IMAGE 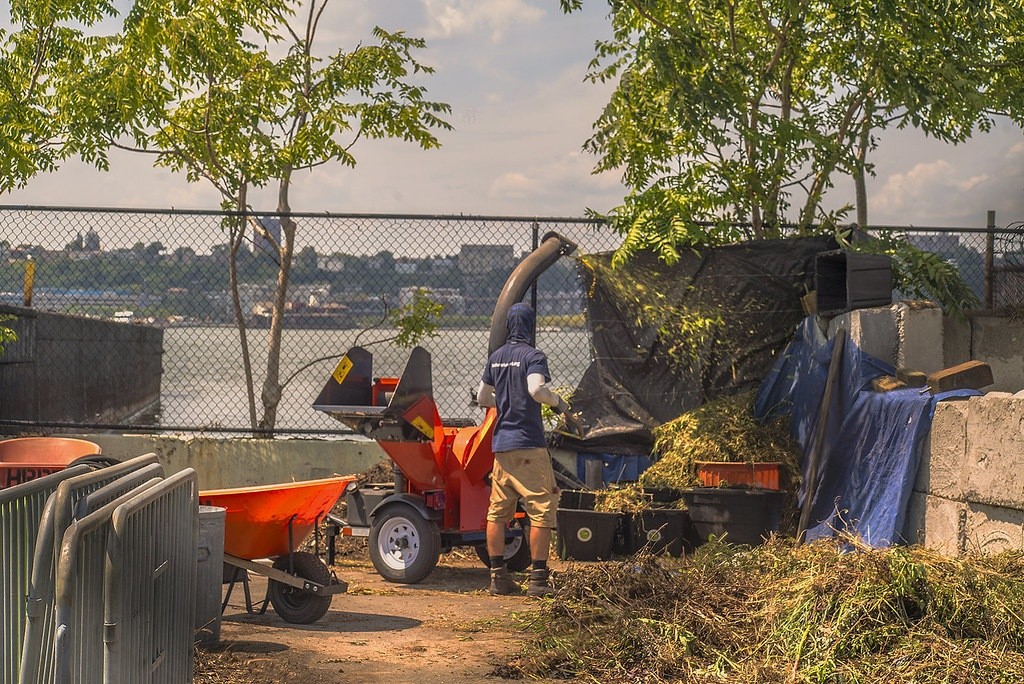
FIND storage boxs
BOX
[555,460,784,562]
[814,249,892,317]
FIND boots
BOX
[528,566,566,597]
[489,564,522,594]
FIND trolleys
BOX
[196,474,357,624]
[0,435,102,491]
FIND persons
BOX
[476,303,585,597]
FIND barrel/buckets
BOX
[624,502,686,558]
[557,489,624,562]
[193,506,226,649]
[681,485,788,549]
[642,486,679,503]
